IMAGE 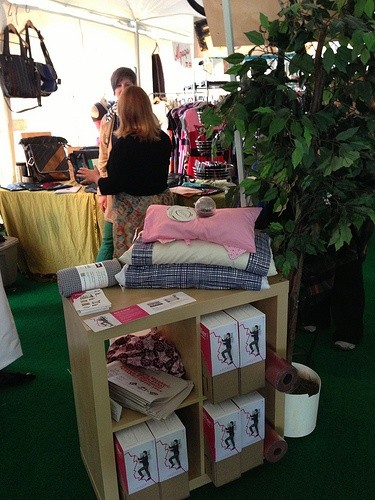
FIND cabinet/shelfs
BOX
[64,279,290,500]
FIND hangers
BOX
[150,92,214,109]
[0,20,44,47]
[152,43,160,55]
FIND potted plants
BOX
[206,1,375,437]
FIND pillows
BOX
[115,205,278,290]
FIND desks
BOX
[0,188,114,282]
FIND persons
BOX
[96,67,137,262]
[0,273,36,387]
[137,450,151,481]
[97,315,114,327]
[249,409,259,437]
[76,86,172,259]
[224,421,236,451]
[168,439,181,470]
[91,94,115,146]
[249,325,260,357]
[221,333,234,365]
[300,75,375,351]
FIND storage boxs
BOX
[115,303,266,500]
[0,236,19,286]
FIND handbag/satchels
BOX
[1,26,45,113]
[24,24,61,98]
[18,136,73,183]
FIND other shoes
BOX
[0,370,36,387]
